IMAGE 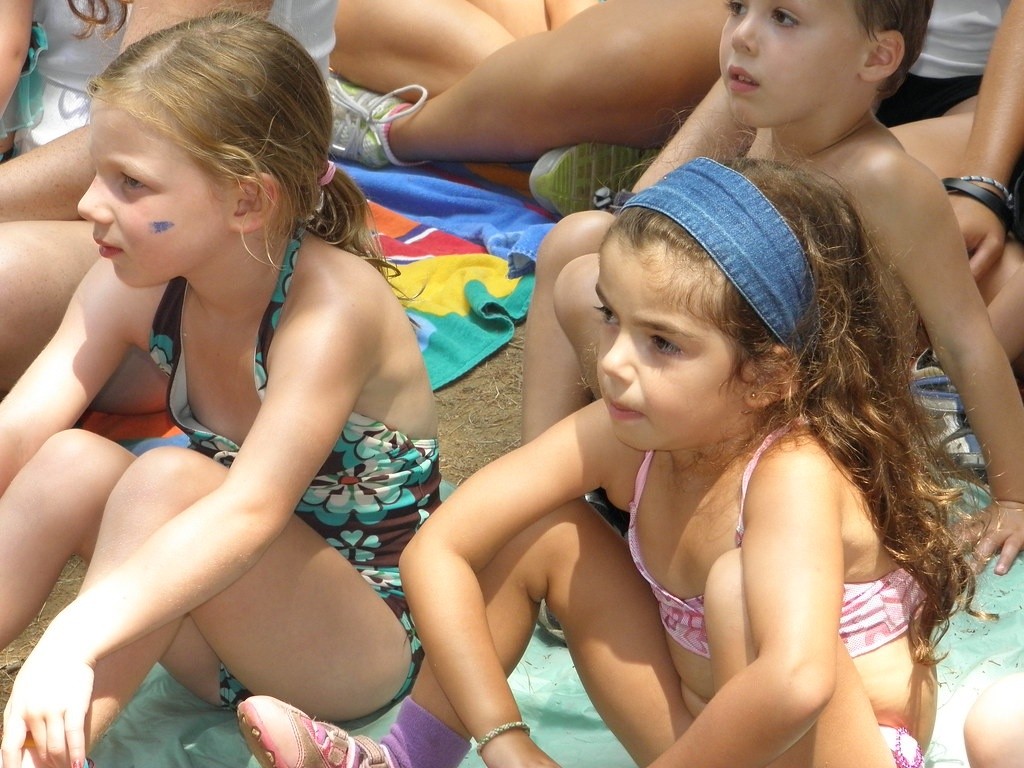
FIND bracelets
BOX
[476,722,531,757]
[940,177,1015,232]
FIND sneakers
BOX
[236,693,393,768]
[529,140,684,218]
[285,73,427,169]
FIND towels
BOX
[63,470,1024,768]
[318,151,571,403]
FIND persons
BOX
[3,0,1024,418]
[227,154,954,768]
[0,15,441,768]
[961,673,1024,768]
[522,0,1023,647]
